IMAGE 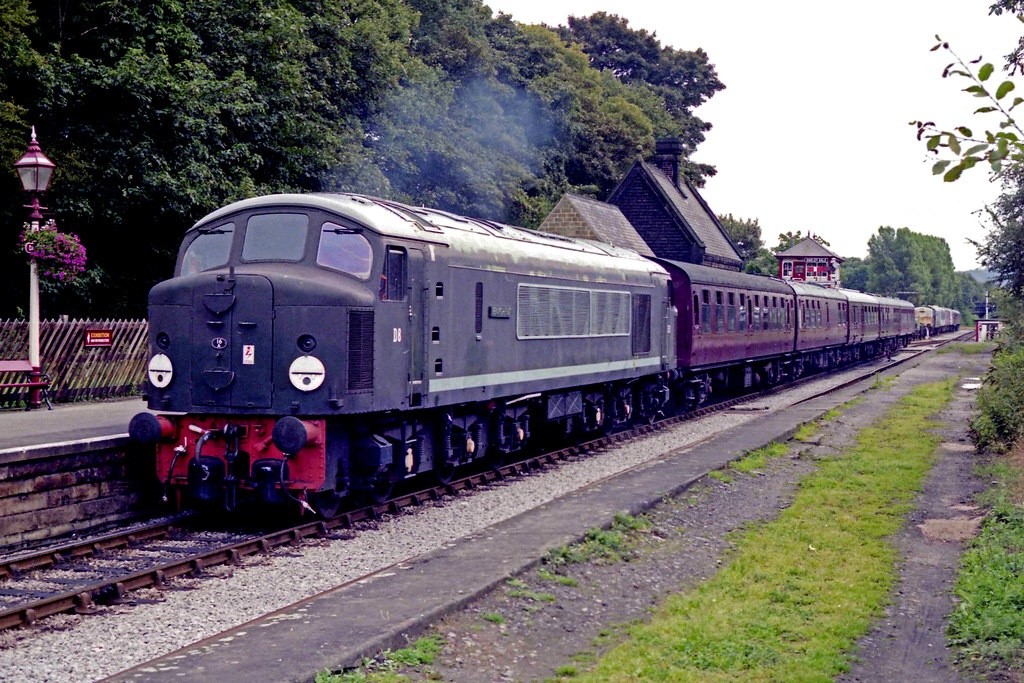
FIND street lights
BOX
[13,125,56,410]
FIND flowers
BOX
[15,218,102,305]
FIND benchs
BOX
[0,360,54,410]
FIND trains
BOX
[116,192,960,530]
